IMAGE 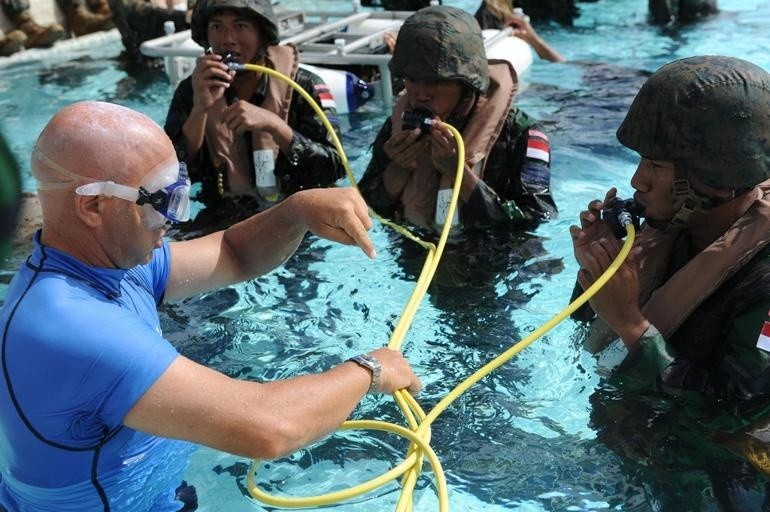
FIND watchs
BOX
[345,354,383,396]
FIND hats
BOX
[387,6,489,92]
[190,1,282,46]
[617,55,770,220]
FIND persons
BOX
[569,54,770,512]
[472,0,565,64]
[55,0,119,38]
[165,1,347,230]
[356,5,559,238]
[108,0,193,60]
[0,0,63,56]
[1,101,423,512]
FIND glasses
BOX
[75,159,192,223]
